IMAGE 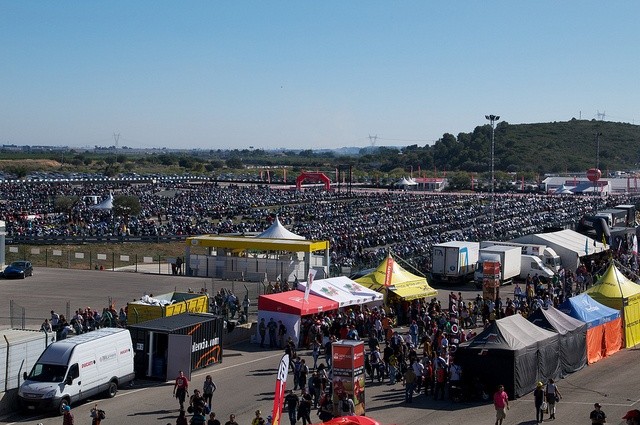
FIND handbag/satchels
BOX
[555,385,560,402]
[543,396,548,414]
[540,390,547,410]
[187,396,195,413]
[416,363,424,374]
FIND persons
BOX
[41,287,251,339]
[267,415,271,424]
[251,410,265,425]
[308,297,449,398]
[493,385,509,424]
[63,405,74,424]
[176,410,188,424]
[589,403,607,425]
[202,375,216,409]
[173,371,189,408]
[278,320,287,348]
[208,412,221,425]
[338,391,355,415]
[283,336,335,425]
[544,377,563,420]
[0,179,635,276]
[259,317,267,346]
[450,264,593,328]
[225,414,239,424]
[189,406,206,425]
[533,381,546,424]
[189,388,205,414]
[621,409,640,425]
[267,317,277,347]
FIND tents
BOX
[578,259,640,350]
[297,276,384,314]
[352,247,438,324]
[525,303,587,374]
[256,288,338,346]
[555,291,622,365]
[458,314,558,400]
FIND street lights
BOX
[485,114,500,239]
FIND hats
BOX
[63,406,70,411]
[622,410,640,419]
[347,309,352,311]
[536,382,543,386]
[194,389,200,393]
[266,416,271,419]
[303,394,312,400]
[594,403,601,407]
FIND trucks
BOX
[522,255,554,280]
[595,209,626,227]
[481,241,562,273]
[431,240,480,279]
[474,245,522,290]
[18,328,135,417]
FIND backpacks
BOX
[341,399,350,412]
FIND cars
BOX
[4,260,34,281]
